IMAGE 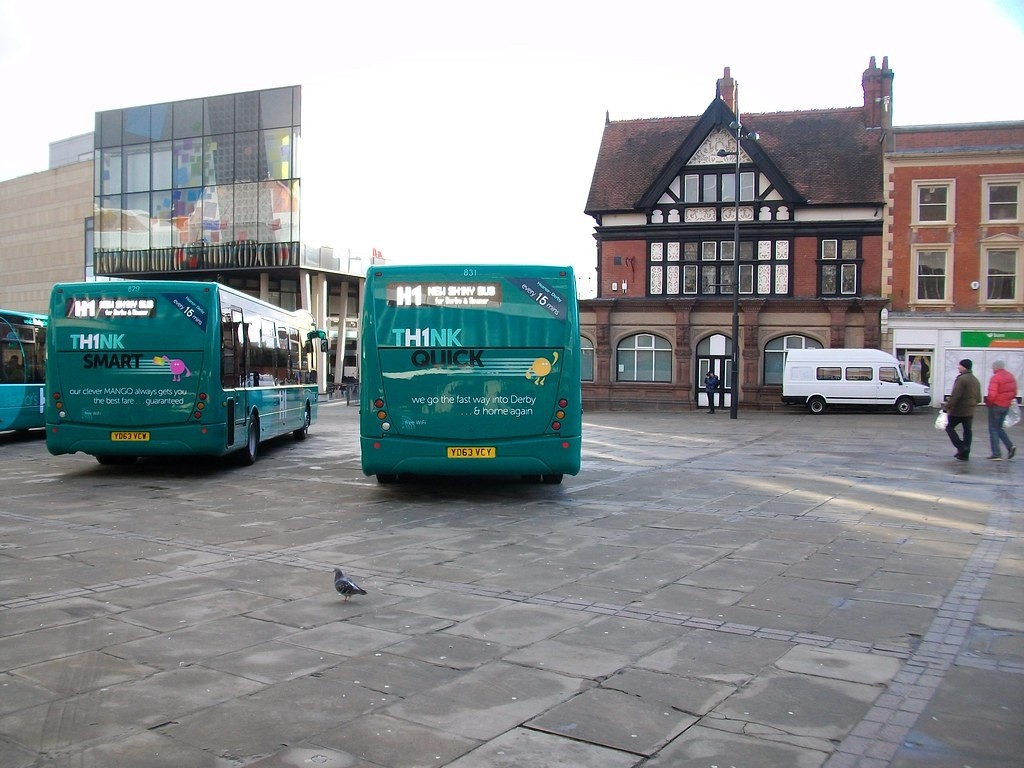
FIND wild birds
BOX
[332,566,368,603]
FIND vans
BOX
[781,346,932,416]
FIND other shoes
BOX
[1008,445,1016,459]
[954,453,969,462]
[707,410,714,414]
[986,456,1003,461]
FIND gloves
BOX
[943,409,947,413]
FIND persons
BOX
[704,371,717,414]
[987,360,1016,460]
[943,359,982,461]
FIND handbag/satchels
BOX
[935,410,948,430]
[1003,399,1021,427]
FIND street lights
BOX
[718,79,756,420]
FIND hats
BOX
[994,360,1005,368]
[708,371,713,373]
[959,359,972,370]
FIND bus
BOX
[356,265,584,485]
[0,308,47,435]
[44,280,330,466]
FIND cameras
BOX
[706,373,710,376]
